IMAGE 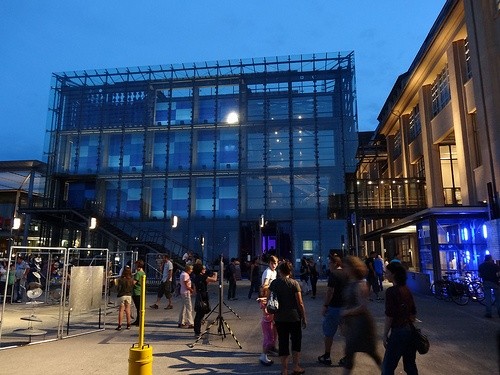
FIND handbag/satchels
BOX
[414,328,429,355]
[266,281,280,314]
[197,300,211,314]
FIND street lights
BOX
[169,215,179,261]
[199,235,206,267]
[87,217,97,247]
[257,213,265,262]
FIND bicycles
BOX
[429,270,499,307]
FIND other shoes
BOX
[228,297,239,301]
[131,321,139,325]
[188,325,194,329]
[368,298,373,302]
[117,325,121,330]
[311,296,315,298]
[127,325,131,329]
[270,348,279,356]
[318,353,332,366]
[178,323,187,328]
[484,314,492,318]
[150,304,158,309]
[195,333,205,338]
[291,367,305,375]
[337,357,346,365]
[375,297,382,302]
[260,356,274,365]
[166,305,173,310]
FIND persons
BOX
[327,249,343,260]
[342,256,382,375]
[317,256,354,368]
[248,260,261,299]
[266,260,308,375]
[224,257,241,301]
[190,263,217,340]
[114,268,133,330]
[300,256,312,295]
[182,250,198,266]
[379,262,420,375]
[275,257,295,280]
[308,256,319,299]
[178,264,194,328]
[259,284,276,366]
[363,251,415,301]
[0,255,64,303]
[263,255,280,357]
[479,254,500,319]
[132,259,145,327]
[150,255,174,310]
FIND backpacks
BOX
[224,266,232,280]
